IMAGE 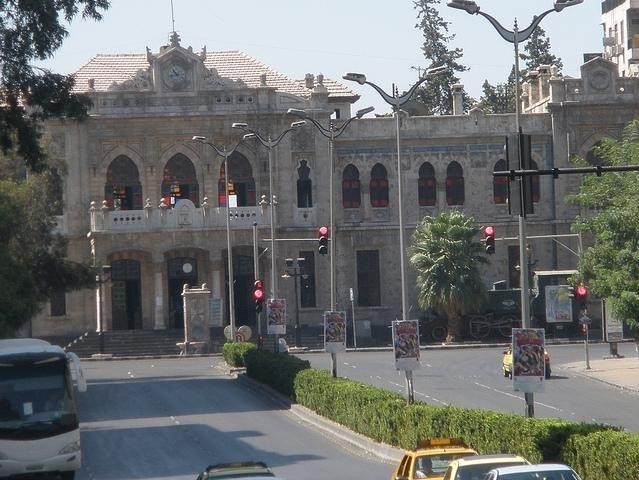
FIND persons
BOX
[279,334,289,354]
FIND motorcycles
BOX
[417,306,447,342]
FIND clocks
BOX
[167,65,186,84]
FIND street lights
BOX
[341,65,449,401]
[281,258,311,350]
[287,107,374,378]
[191,132,258,342]
[231,120,308,354]
[445,0,585,420]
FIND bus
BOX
[0,338,88,480]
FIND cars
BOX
[389,438,583,480]
[196,461,277,480]
[502,341,551,379]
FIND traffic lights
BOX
[574,284,587,300]
[484,225,495,255]
[253,279,265,312]
[319,226,328,254]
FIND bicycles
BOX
[469,310,521,340]
